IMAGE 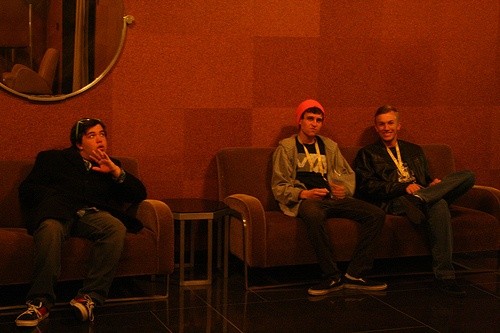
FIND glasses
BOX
[76,118,104,139]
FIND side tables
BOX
[165,198,228,287]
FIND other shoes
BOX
[397,194,427,224]
[436,279,467,297]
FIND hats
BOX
[296,99,325,125]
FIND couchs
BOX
[215,147,500,290]
[0,156,175,310]
[2,48,59,95]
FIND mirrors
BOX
[0,0,134,102]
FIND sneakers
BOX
[14,300,49,326]
[69,294,95,321]
[341,273,387,290]
[308,278,343,296]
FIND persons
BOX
[352,105,476,295]
[14,117,147,326]
[271,99,388,295]
